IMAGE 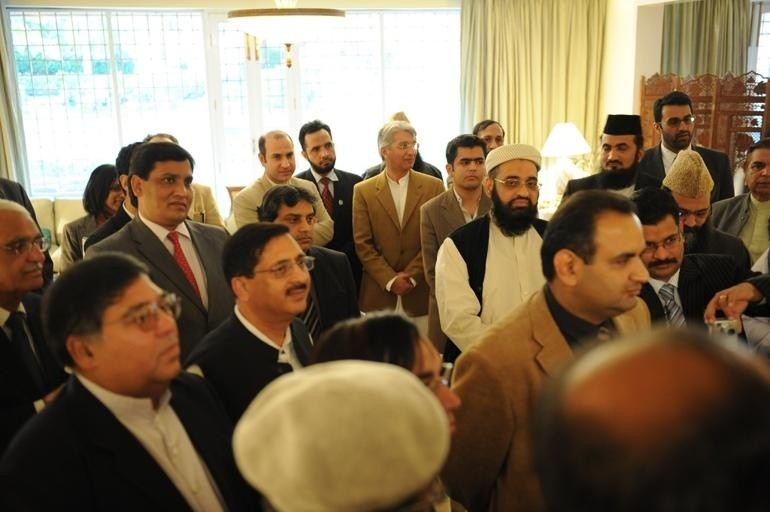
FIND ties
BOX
[319,177,335,218]
[595,326,615,346]
[166,231,203,304]
[296,293,323,346]
[658,283,688,331]
[3,311,42,381]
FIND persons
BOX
[0,92,769,512]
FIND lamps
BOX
[229,0,347,69]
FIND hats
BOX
[485,144,542,175]
[603,115,641,134]
[663,150,714,197]
[233,360,451,512]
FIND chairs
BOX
[30,198,89,273]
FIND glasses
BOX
[643,231,681,250]
[112,185,124,191]
[103,293,181,331]
[657,116,695,127]
[248,256,314,277]
[389,144,418,150]
[278,217,318,225]
[679,206,713,218]
[494,177,542,190]
[426,362,455,389]
[1,236,51,256]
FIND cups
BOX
[708,320,738,352]
